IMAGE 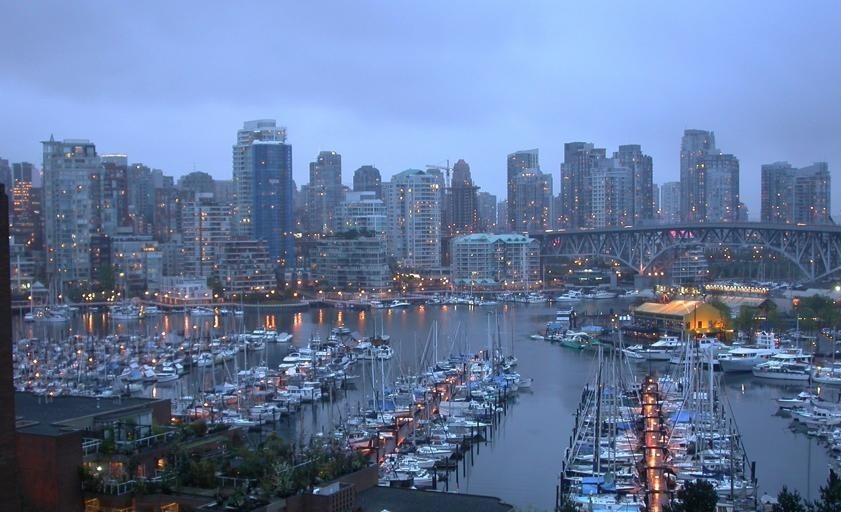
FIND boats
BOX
[717,328,783,374]
[498,280,548,303]
[370,300,385,309]
[13,306,322,430]
[752,349,841,454]
[554,284,638,301]
[421,280,497,306]
[389,300,412,308]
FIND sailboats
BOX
[542,300,760,512]
[323,306,532,488]
